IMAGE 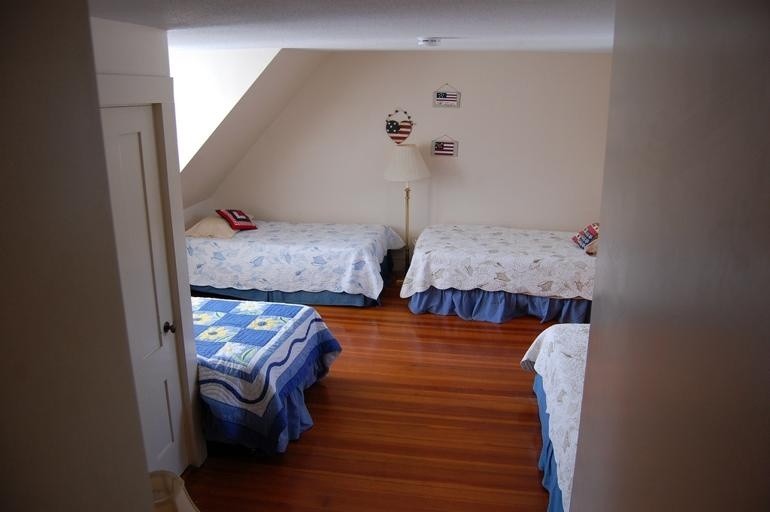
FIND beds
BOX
[186,219,406,307]
[191,295,342,452]
[520,322,590,512]
[400,224,597,324]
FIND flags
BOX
[436,92,457,105]
[434,142,454,155]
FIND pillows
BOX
[184,213,255,238]
[571,223,599,249]
[585,238,598,255]
[215,208,257,229]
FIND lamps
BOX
[385,144,431,284]
